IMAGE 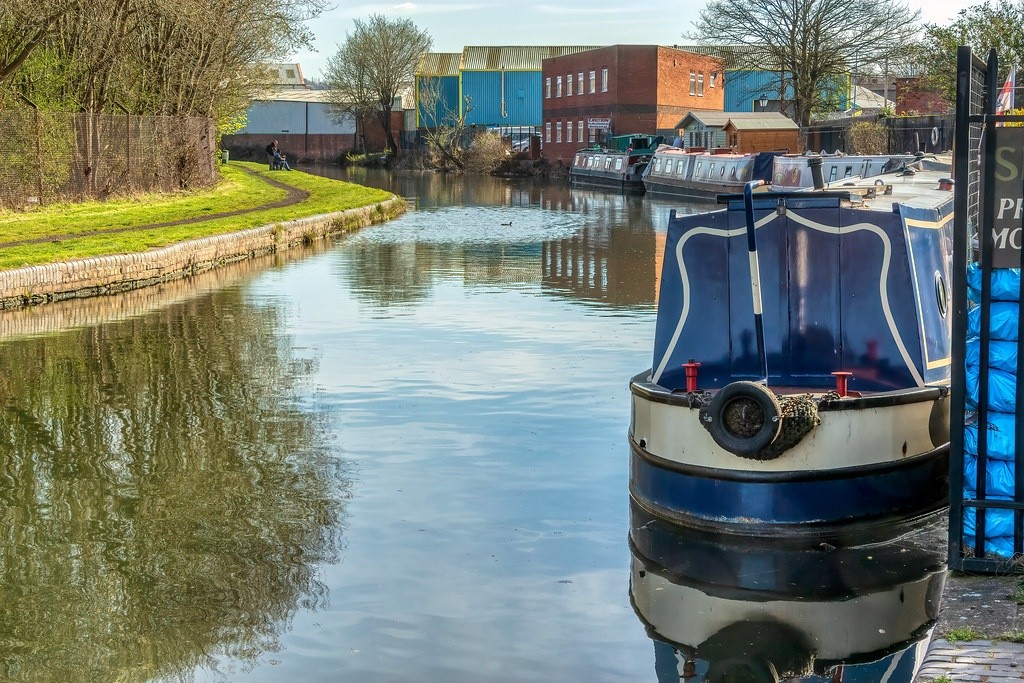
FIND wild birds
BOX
[501,221,513,227]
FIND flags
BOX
[995,72,1013,120]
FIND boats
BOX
[565,133,951,683]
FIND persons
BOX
[266,139,279,170]
[274,149,293,171]
